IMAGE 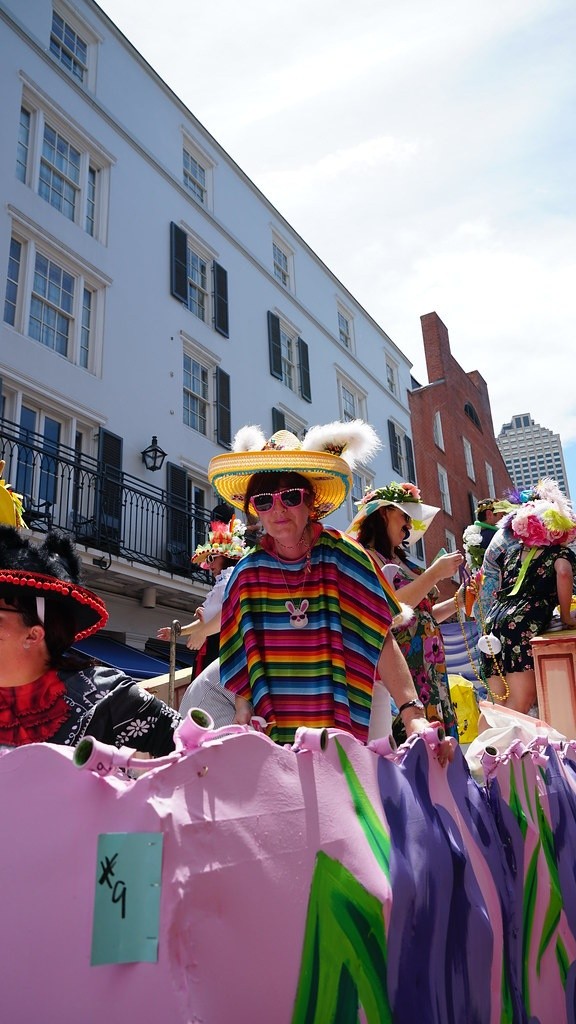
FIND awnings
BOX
[70,632,179,680]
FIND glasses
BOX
[205,554,219,564]
[249,489,309,513]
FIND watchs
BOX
[400,699,424,712]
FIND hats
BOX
[0,460,109,643]
[345,481,441,549]
[207,418,379,518]
[191,514,250,564]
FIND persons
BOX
[178,658,237,741]
[156,522,246,680]
[218,430,454,771]
[345,482,477,744]
[0,525,181,772]
[211,502,260,533]
[466,480,576,715]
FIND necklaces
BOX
[454,583,509,699]
[275,548,309,628]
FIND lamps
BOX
[141,436,168,472]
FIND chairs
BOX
[68,513,120,556]
[14,491,54,532]
[167,541,207,579]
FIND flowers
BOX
[353,482,422,510]
[462,525,488,570]
